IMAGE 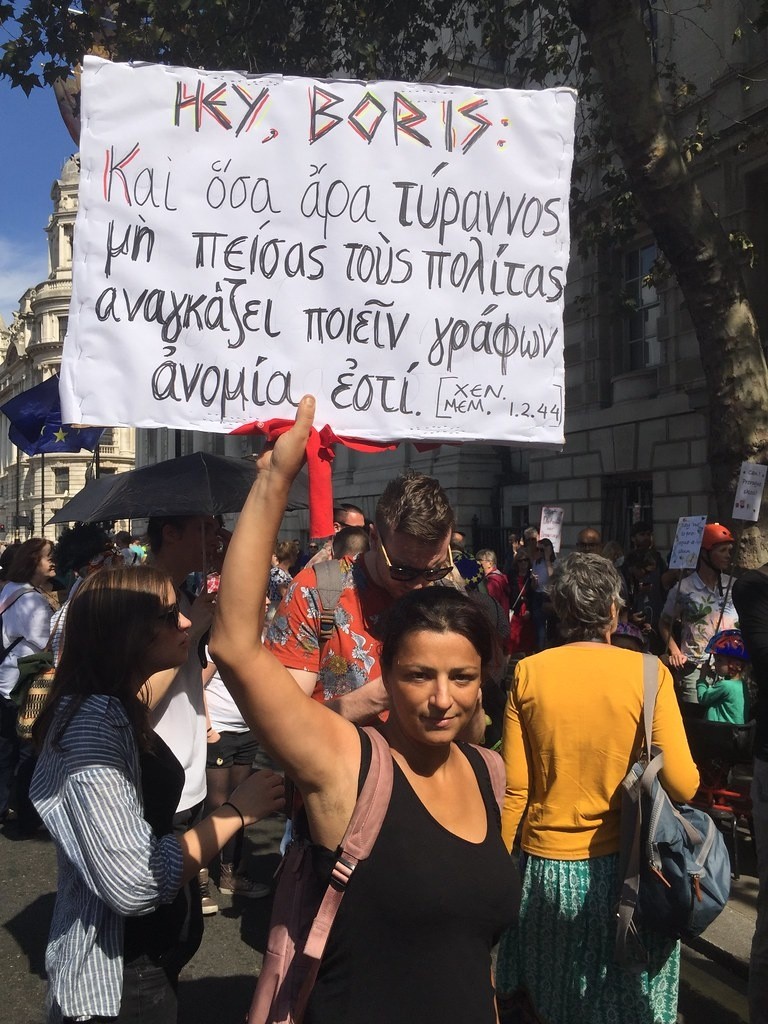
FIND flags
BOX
[0,370,107,458]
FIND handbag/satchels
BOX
[14,608,64,741]
[540,601,557,617]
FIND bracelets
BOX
[222,802,244,832]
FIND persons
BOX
[733,564,768,1024]
[450,520,758,724]
[500,552,703,1024]
[207,393,522,1024]
[0,503,372,913]
[29,562,288,1024]
[275,468,487,746]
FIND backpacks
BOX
[612,653,731,974]
[244,725,506,1024]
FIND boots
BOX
[219,862,272,900]
[198,868,219,914]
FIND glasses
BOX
[375,528,453,581]
[517,558,529,563]
[642,567,654,576]
[525,537,537,542]
[575,543,602,551]
[536,547,544,552]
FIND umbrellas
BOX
[42,449,349,528]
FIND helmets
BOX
[702,523,735,550]
[704,629,752,662]
[612,620,644,651]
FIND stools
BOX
[705,808,742,881]
